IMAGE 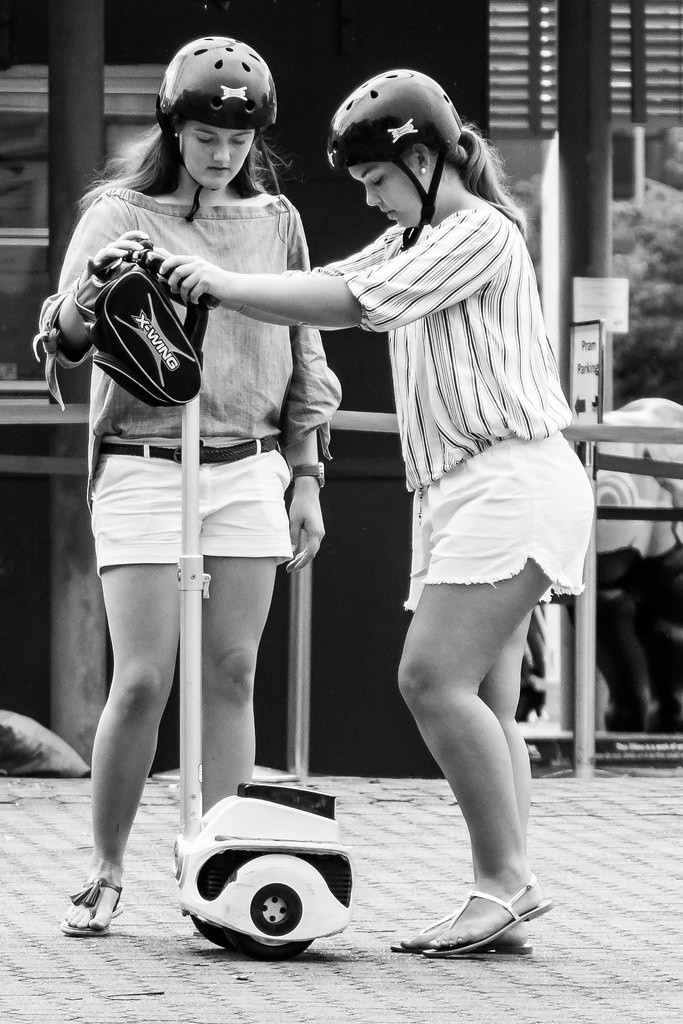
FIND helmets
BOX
[156,35,277,128]
[326,68,463,170]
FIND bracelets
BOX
[237,305,247,313]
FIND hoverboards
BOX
[74,240,354,963]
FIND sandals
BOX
[390,908,533,955]
[61,877,124,935]
[423,873,554,962]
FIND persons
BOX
[133,68,595,958]
[34,36,342,936]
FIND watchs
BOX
[290,462,325,488]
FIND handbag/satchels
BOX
[74,252,209,406]
